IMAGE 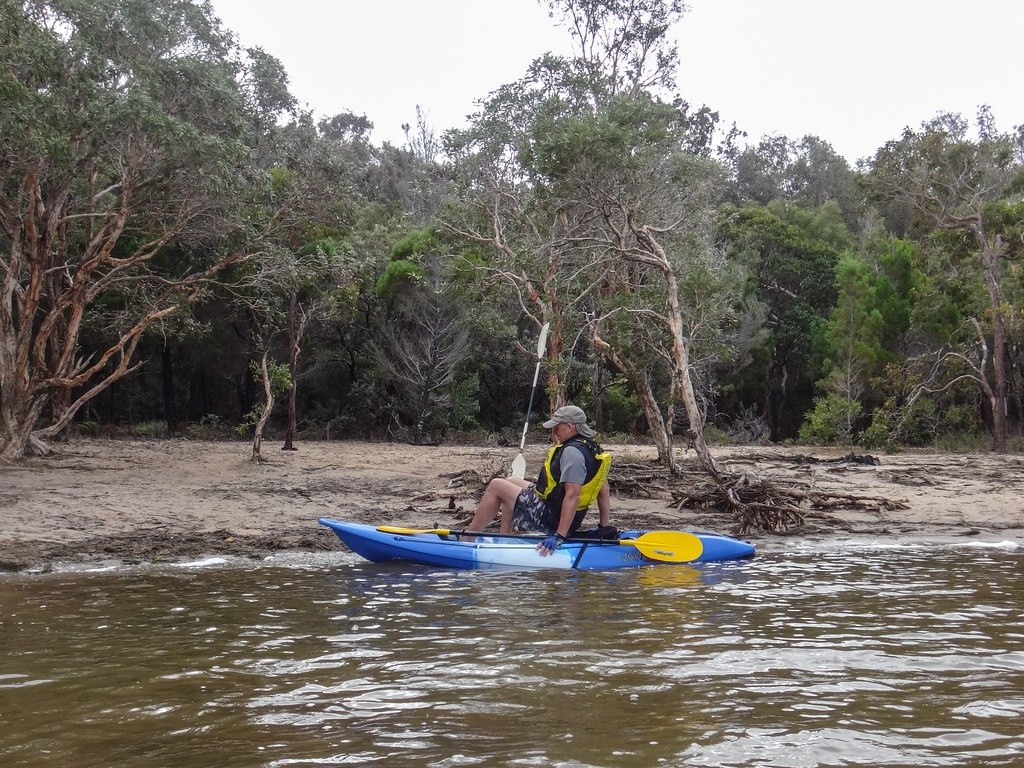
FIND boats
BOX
[318,518,757,570]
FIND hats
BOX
[542,405,597,438]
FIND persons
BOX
[433,405,612,558]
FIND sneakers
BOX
[433,523,459,542]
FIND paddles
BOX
[506,321,551,480]
[373,524,705,563]
